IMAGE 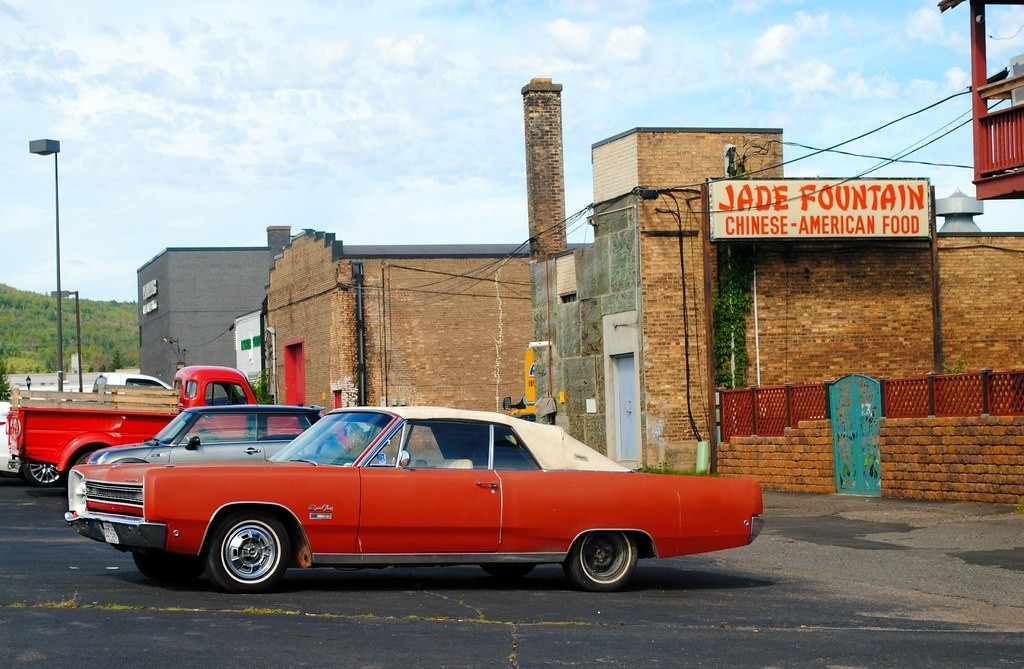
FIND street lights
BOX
[28,137,72,392]
[48,290,88,391]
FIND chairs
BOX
[435,459,474,469]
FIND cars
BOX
[85,404,353,474]
[64,406,768,598]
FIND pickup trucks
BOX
[2,366,310,487]
[0,372,174,487]
